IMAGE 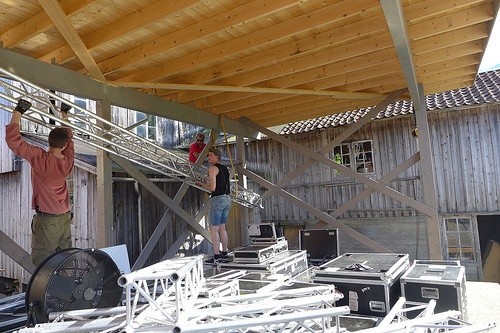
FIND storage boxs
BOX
[248,223,288,256]
[313,252,409,316]
[482,240,500,282]
[400,263,468,331]
[233,244,273,264]
[298,228,340,261]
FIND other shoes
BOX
[204,254,227,264]
[222,252,230,262]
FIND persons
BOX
[5,98,74,264]
[194,148,231,264]
[188,133,209,168]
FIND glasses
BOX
[196,139,203,142]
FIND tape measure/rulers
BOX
[412,128,418,136]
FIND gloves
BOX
[15,98,32,115]
[60,102,72,114]
